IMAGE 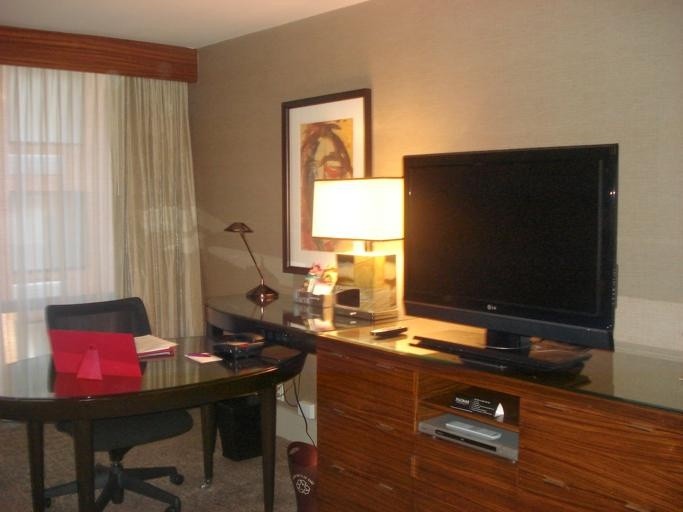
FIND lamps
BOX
[311,174,405,322]
[224,220,277,298]
[249,298,276,322]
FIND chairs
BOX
[46,297,194,512]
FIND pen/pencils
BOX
[188,352,210,357]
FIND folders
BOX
[136,347,176,359]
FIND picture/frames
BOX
[279,88,374,276]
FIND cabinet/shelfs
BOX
[312,316,683,512]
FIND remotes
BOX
[370,325,408,336]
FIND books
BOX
[134,334,179,360]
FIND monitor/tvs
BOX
[402,143,618,375]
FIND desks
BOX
[0,335,306,512]
[202,290,409,485]
